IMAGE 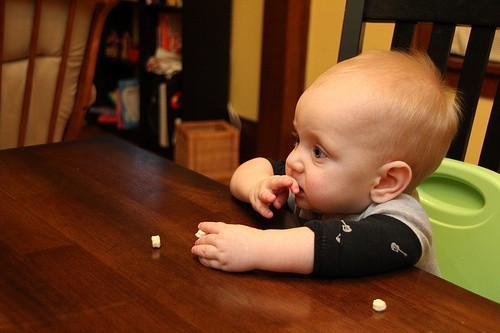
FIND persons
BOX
[191,50,463,279]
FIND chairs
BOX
[337,1,499,174]
[0,0,117,148]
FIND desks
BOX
[1,134,500,333]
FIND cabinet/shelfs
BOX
[83,0,234,158]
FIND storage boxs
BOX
[174,121,240,182]
